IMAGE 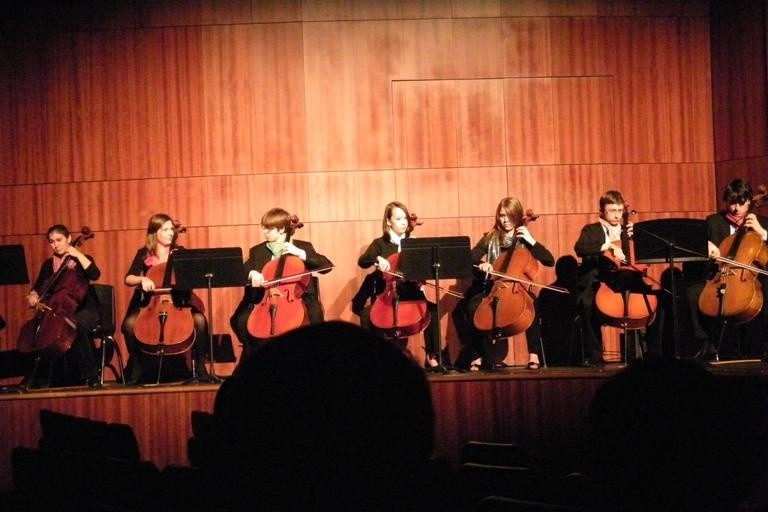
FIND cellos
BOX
[476,207,543,337]
[133,220,196,356]
[370,212,431,338]
[595,204,657,328]
[18,225,95,358]
[698,184,768,325]
[248,214,310,340]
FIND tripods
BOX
[191,273,224,386]
[423,263,458,377]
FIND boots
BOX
[127,342,144,386]
[195,351,221,385]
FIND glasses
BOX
[261,225,275,234]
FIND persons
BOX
[24,224,102,391]
[574,190,664,369]
[359,202,450,375]
[120,213,222,388]
[229,208,334,345]
[589,351,747,512]
[697,177,768,362]
[213,322,435,512]
[468,197,555,373]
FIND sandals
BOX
[527,356,540,370]
[470,360,481,372]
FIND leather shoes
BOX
[579,357,600,367]
[88,378,102,389]
[424,358,447,373]
